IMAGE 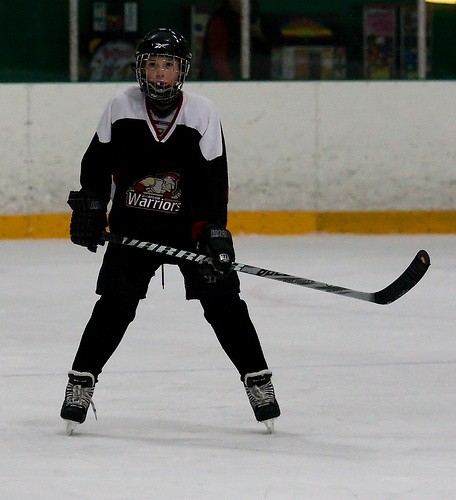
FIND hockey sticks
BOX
[100,231,431,308]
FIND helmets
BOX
[135,27,192,106]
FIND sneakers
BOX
[59,369,98,436]
[241,368,282,435]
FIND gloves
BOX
[208,228,236,272]
[67,190,109,253]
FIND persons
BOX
[59,29,282,425]
